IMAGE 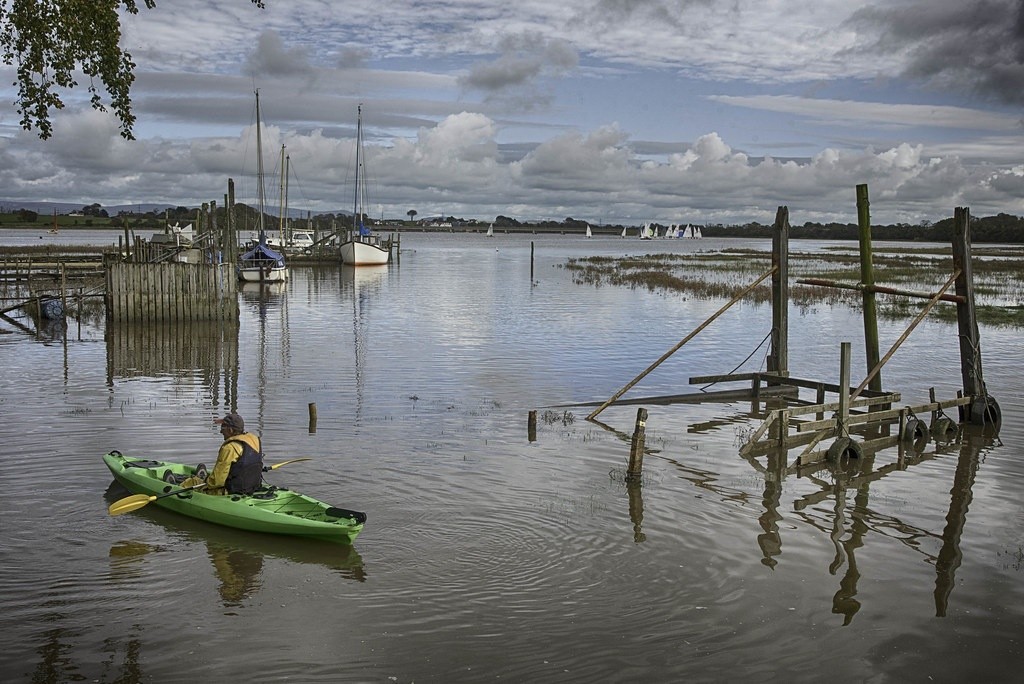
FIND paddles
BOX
[109,457,317,516]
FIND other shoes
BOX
[197,463,208,477]
[163,469,176,484]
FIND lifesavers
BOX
[904,420,931,440]
[826,437,863,464]
[933,417,959,435]
[970,397,1002,425]
[824,425,1001,480]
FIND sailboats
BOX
[653,226,658,239]
[340,103,391,266]
[236,88,289,283]
[640,222,652,240]
[694,227,702,239]
[672,224,679,239]
[586,225,592,238]
[682,223,692,239]
[622,227,626,239]
[664,224,673,239]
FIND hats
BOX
[214,414,244,431]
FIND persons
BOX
[163,414,263,495]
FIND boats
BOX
[102,449,367,546]
[256,232,314,252]
[486,222,494,237]
